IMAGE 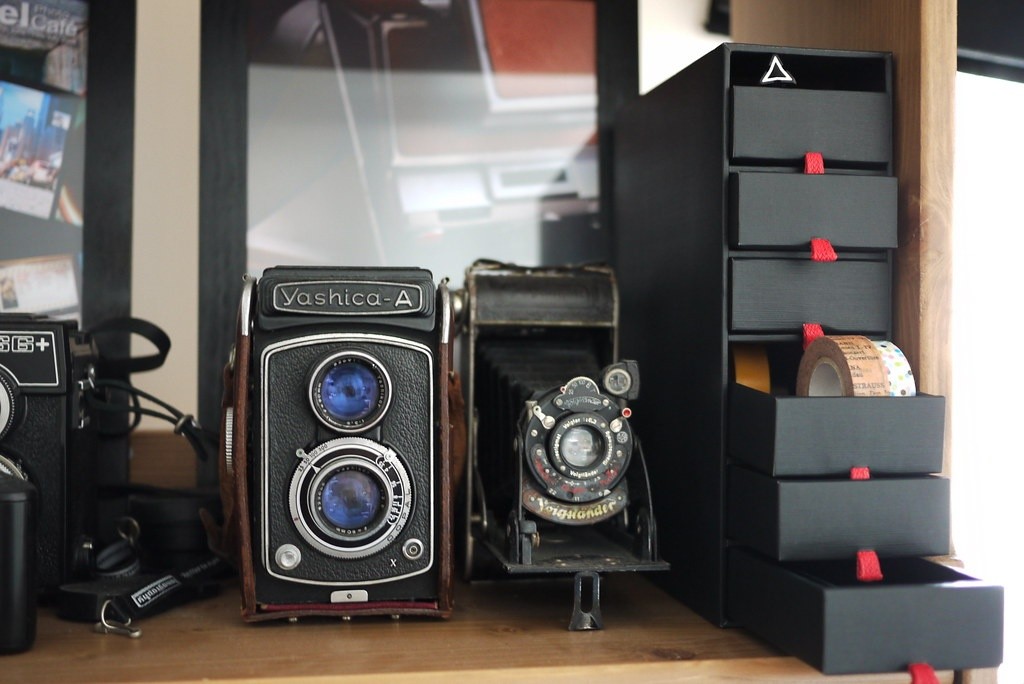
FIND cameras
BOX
[248,264,440,602]
[0,309,105,654]
[455,259,672,630]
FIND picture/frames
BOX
[195,0,641,486]
[0,0,137,488]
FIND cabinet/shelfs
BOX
[614,41,1004,677]
